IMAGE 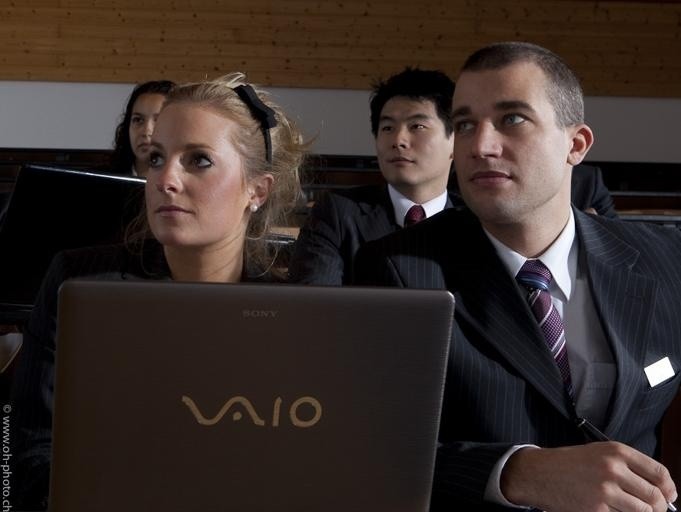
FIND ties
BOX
[403,205,427,231]
[514,260,578,415]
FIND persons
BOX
[9,41,681,512]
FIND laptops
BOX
[46,279,457,512]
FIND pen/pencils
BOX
[574,416,677,512]
